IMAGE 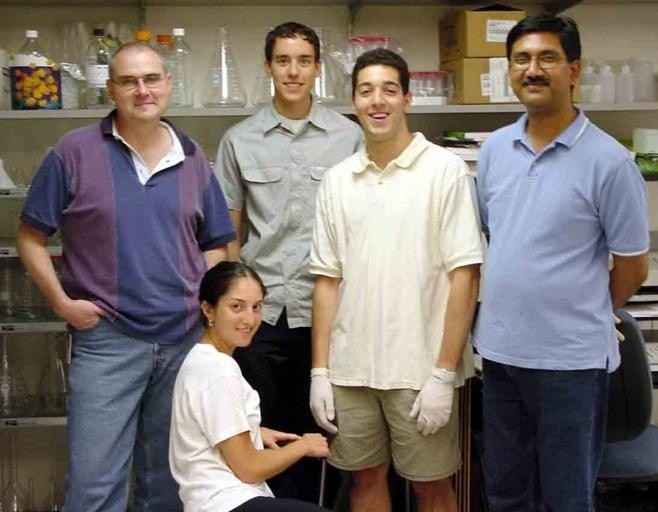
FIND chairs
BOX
[596,307,658,511]
[269,436,328,505]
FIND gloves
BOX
[311,368,338,435]
[410,367,456,438]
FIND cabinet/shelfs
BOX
[1,101,656,428]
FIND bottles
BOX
[155,34,173,78]
[81,27,116,108]
[0,334,69,511]
[581,55,656,104]
[168,27,194,108]
[11,29,51,66]
[203,27,248,109]
[135,29,155,48]
[310,27,336,107]
[252,28,276,107]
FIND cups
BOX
[1,265,48,318]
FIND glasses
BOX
[509,55,567,71]
[111,73,165,90]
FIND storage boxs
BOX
[439,1,527,104]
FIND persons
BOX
[466,11,651,512]
[12,39,237,511]
[166,260,334,511]
[301,47,484,512]
[209,18,367,511]
